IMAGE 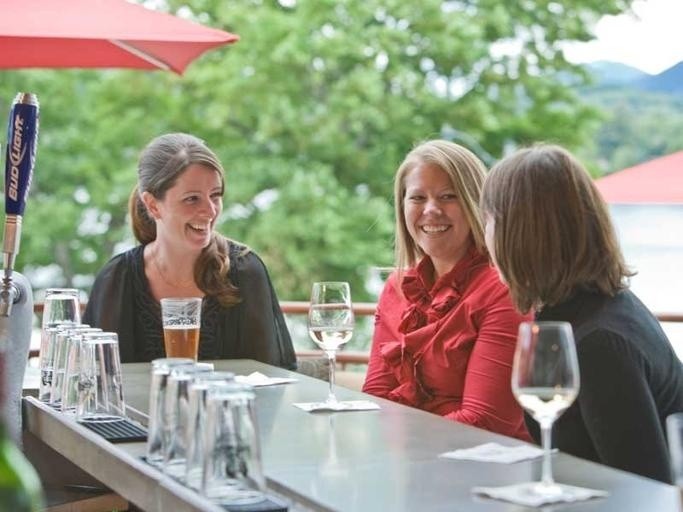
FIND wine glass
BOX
[308,280,357,404]
[506,317,593,494]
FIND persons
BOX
[472,147,683,487]
[354,137,534,444]
[75,128,282,375]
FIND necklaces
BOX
[145,244,201,287]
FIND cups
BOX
[36,285,123,424]
[156,294,204,356]
[141,349,270,508]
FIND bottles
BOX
[0,419,45,511]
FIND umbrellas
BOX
[0,1,241,79]
